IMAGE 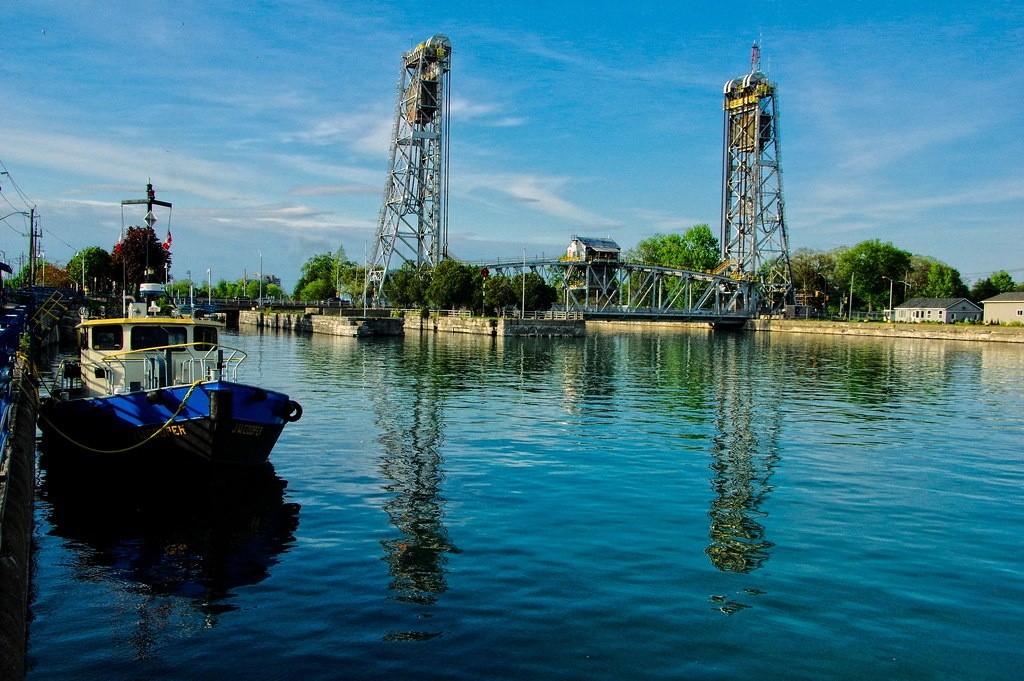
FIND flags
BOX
[162,233,171,249]
[117,231,123,254]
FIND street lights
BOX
[75,250,85,291]
[818,273,826,312]
[242,269,247,298]
[207,268,211,305]
[882,276,893,317]
[186,271,193,308]
[37,252,45,287]
[164,264,168,285]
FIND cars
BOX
[235,296,258,305]
[256,298,275,305]
[322,297,341,305]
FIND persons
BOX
[842,291,849,319]
[814,288,825,309]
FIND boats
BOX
[35,184,291,488]
